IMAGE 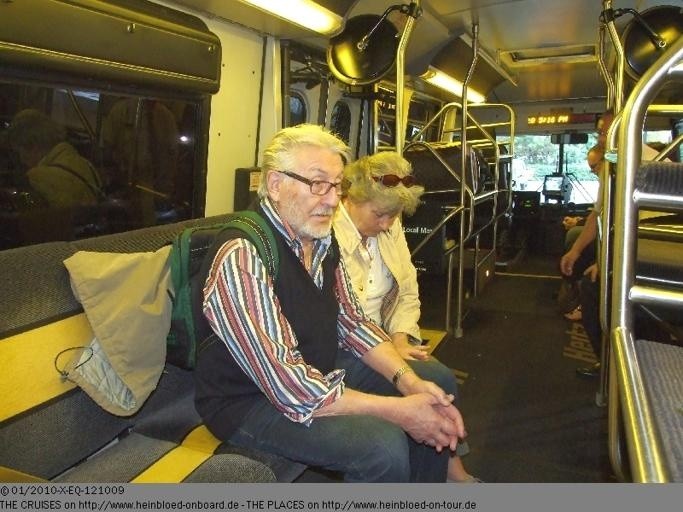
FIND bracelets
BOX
[391,365,413,389]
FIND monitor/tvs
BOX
[543,175,573,202]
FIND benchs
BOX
[0,202,453,482]
[598,166,683,486]
[404,140,509,272]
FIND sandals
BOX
[562,304,582,322]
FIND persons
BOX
[330,151,485,483]
[557,109,677,377]
[0,98,180,223]
[193,122,468,483]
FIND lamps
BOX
[418,63,494,113]
[225,0,358,39]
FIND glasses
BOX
[357,174,420,189]
[590,160,600,168]
[277,169,352,198]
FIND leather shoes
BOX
[576,360,601,378]
